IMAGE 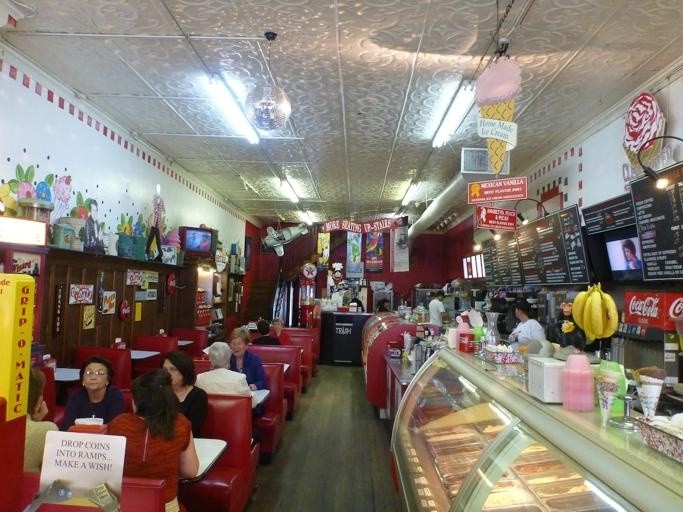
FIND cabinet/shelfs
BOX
[0,242,197,369]
[390,346,683,512]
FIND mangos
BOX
[1,179,17,210]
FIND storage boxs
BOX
[528,357,566,404]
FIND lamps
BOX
[637,136,683,189]
[401,79,477,206]
[196,72,313,227]
[245,32,291,131]
[472,198,549,252]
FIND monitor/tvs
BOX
[603,234,640,274]
[178,226,219,256]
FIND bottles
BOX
[411,329,440,374]
[397,305,429,323]
[388,341,403,359]
[485,311,500,347]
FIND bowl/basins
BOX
[561,354,594,412]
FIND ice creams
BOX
[163,228,181,253]
[620,90,668,174]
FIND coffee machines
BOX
[489,290,537,336]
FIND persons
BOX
[423,291,435,309]
[348,298,366,313]
[229,327,267,417]
[189,232,212,250]
[622,240,642,270]
[428,290,451,336]
[62,357,125,432]
[391,217,406,228]
[145,184,165,262]
[161,350,208,438]
[270,318,293,346]
[250,320,280,346]
[508,300,547,345]
[23,366,60,472]
[193,341,253,399]
[104,368,200,512]
[376,298,390,313]
[85,199,105,254]
[329,270,348,300]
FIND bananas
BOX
[572,283,619,341]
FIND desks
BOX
[384,353,459,426]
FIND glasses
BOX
[82,369,108,376]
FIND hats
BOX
[207,340,233,367]
